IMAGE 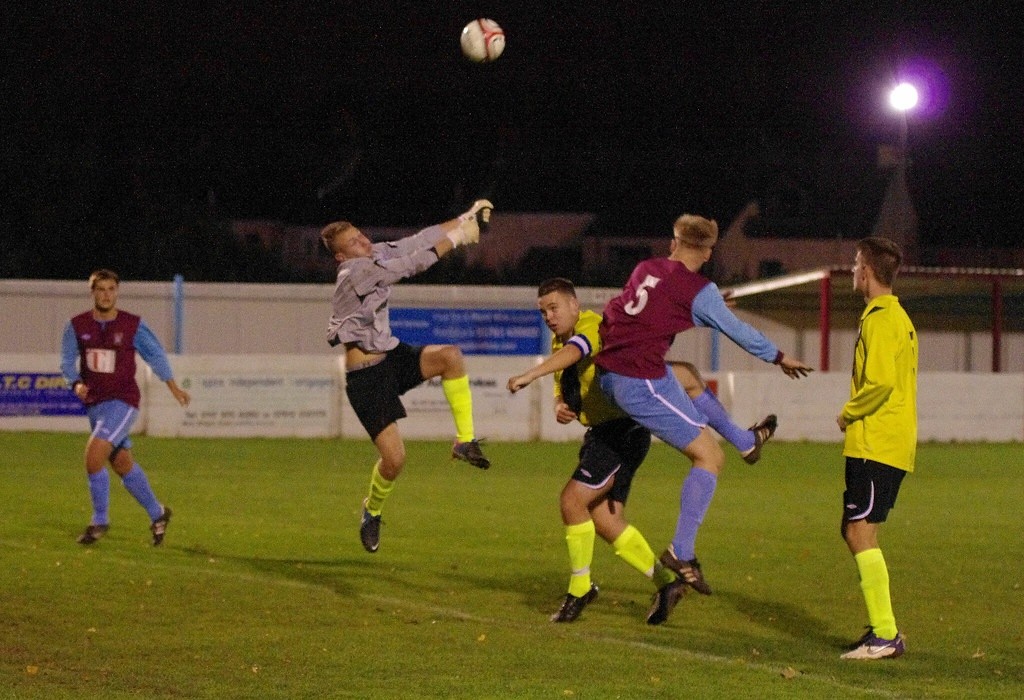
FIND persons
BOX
[835,237,918,658]
[505,279,688,625]
[322,199,494,553]
[596,214,814,594]
[61,270,189,546]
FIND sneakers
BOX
[550,583,597,623]
[76,525,109,545]
[740,415,778,464]
[360,497,386,552]
[660,541,711,595]
[646,576,687,625]
[840,626,905,661]
[149,505,171,546]
[453,436,490,469]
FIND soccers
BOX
[460,17,506,62]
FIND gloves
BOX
[447,220,480,249]
[459,199,494,231]
